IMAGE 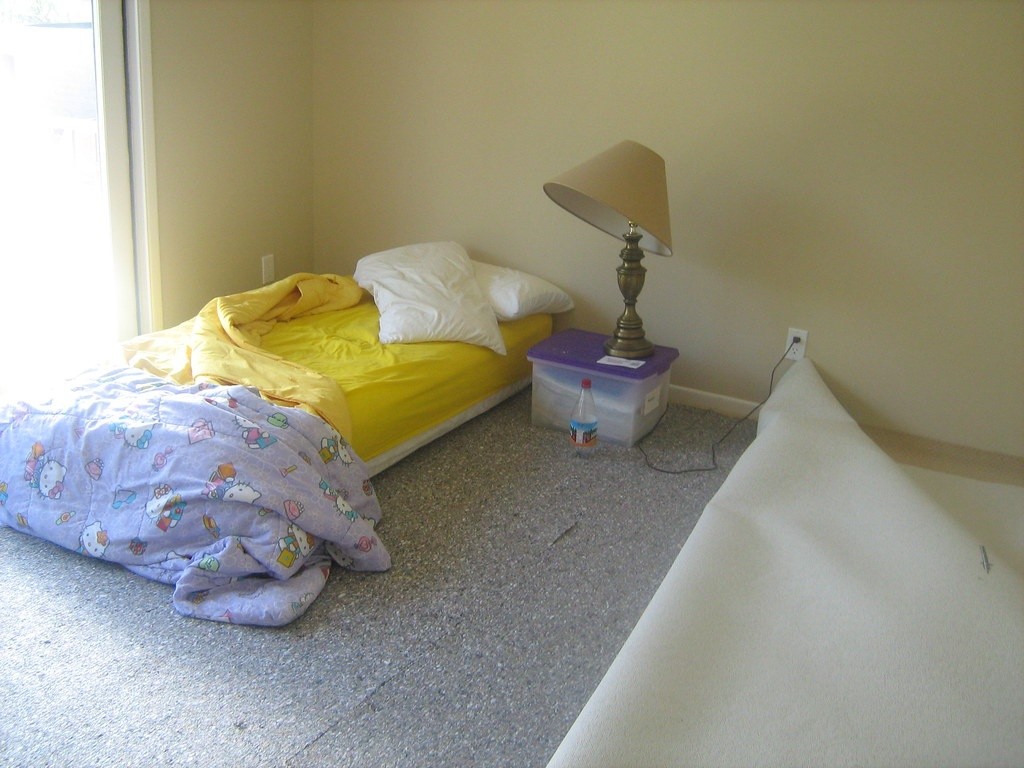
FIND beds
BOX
[0,241,553,628]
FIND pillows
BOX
[354,239,508,356]
[470,259,573,321]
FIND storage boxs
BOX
[527,328,679,446]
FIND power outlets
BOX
[785,327,809,360]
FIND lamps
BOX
[543,138,672,358]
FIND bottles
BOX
[570,378,599,460]
